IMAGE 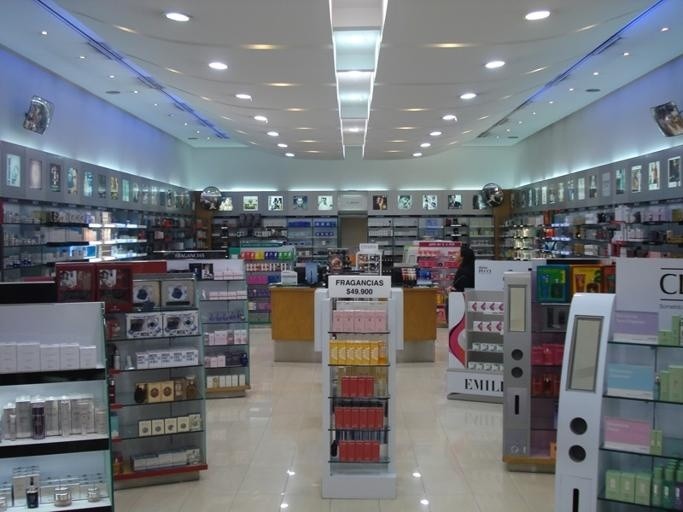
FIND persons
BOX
[294,197,306,210]
[319,197,329,209]
[448,194,462,209]
[453,250,476,292]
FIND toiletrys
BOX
[88,488,99,503]
[32,405,44,441]
[652,458,683,509]
[242,353,248,367]
[114,459,120,476]
[539,274,601,428]
[0,497,6,512]
[26,485,39,508]
[109,379,116,404]
[113,349,120,371]
[55,488,73,507]
[654,376,660,400]
[3,210,44,270]
[134,384,146,403]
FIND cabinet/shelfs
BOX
[239,197,683,328]
[314,275,403,498]
[1,197,252,512]
[446,259,681,510]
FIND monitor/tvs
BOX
[304,262,319,286]
[391,266,417,288]
